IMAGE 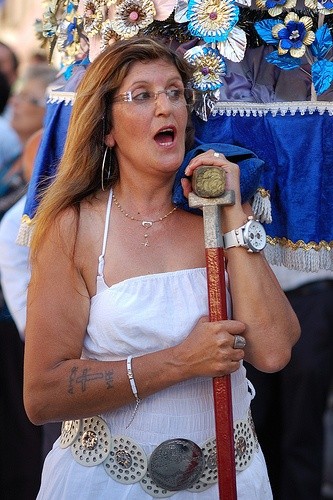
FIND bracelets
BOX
[126,355,143,405]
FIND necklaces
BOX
[112,187,179,248]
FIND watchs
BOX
[224,215,268,253]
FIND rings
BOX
[213,152,221,158]
[234,334,247,349]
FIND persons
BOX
[0,41,19,88]
[23,33,304,500]
[0,62,60,215]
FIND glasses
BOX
[113,88,197,106]
